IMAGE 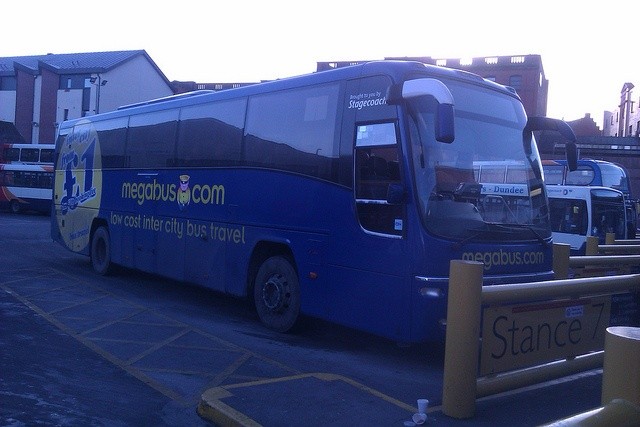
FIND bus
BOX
[50,59,579,333]
[542,158,640,255]
[0,142,55,214]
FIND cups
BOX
[411,413,428,425]
[417,398,429,413]
